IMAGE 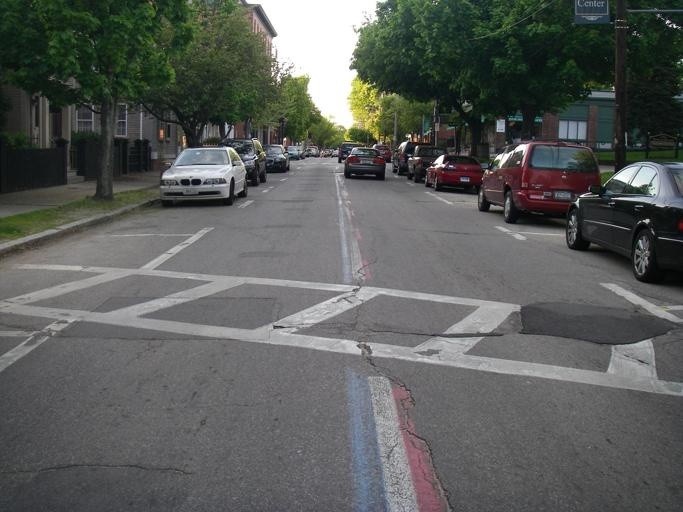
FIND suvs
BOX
[391,141,430,176]
[217,138,267,188]
[338,142,363,163]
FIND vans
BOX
[477,142,602,226]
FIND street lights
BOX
[364,105,381,143]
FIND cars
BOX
[407,146,448,183]
[261,143,292,173]
[286,146,299,161]
[425,154,482,191]
[565,159,683,282]
[344,147,387,180]
[372,144,392,163]
[160,146,249,206]
[293,145,339,160]
